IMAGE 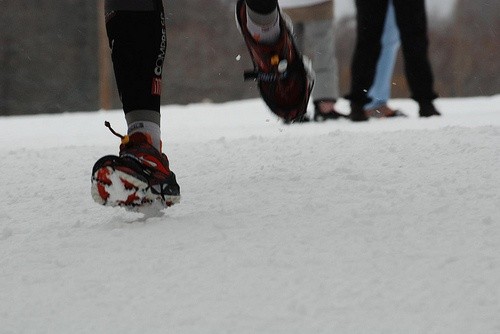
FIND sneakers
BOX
[234,0,314,123]
[91,121,180,209]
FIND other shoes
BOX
[419,106,440,117]
[314,109,345,120]
[351,108,368,122]
[373,109,406,118]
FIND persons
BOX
[91,0,314,212]
[310,0,442,122]
